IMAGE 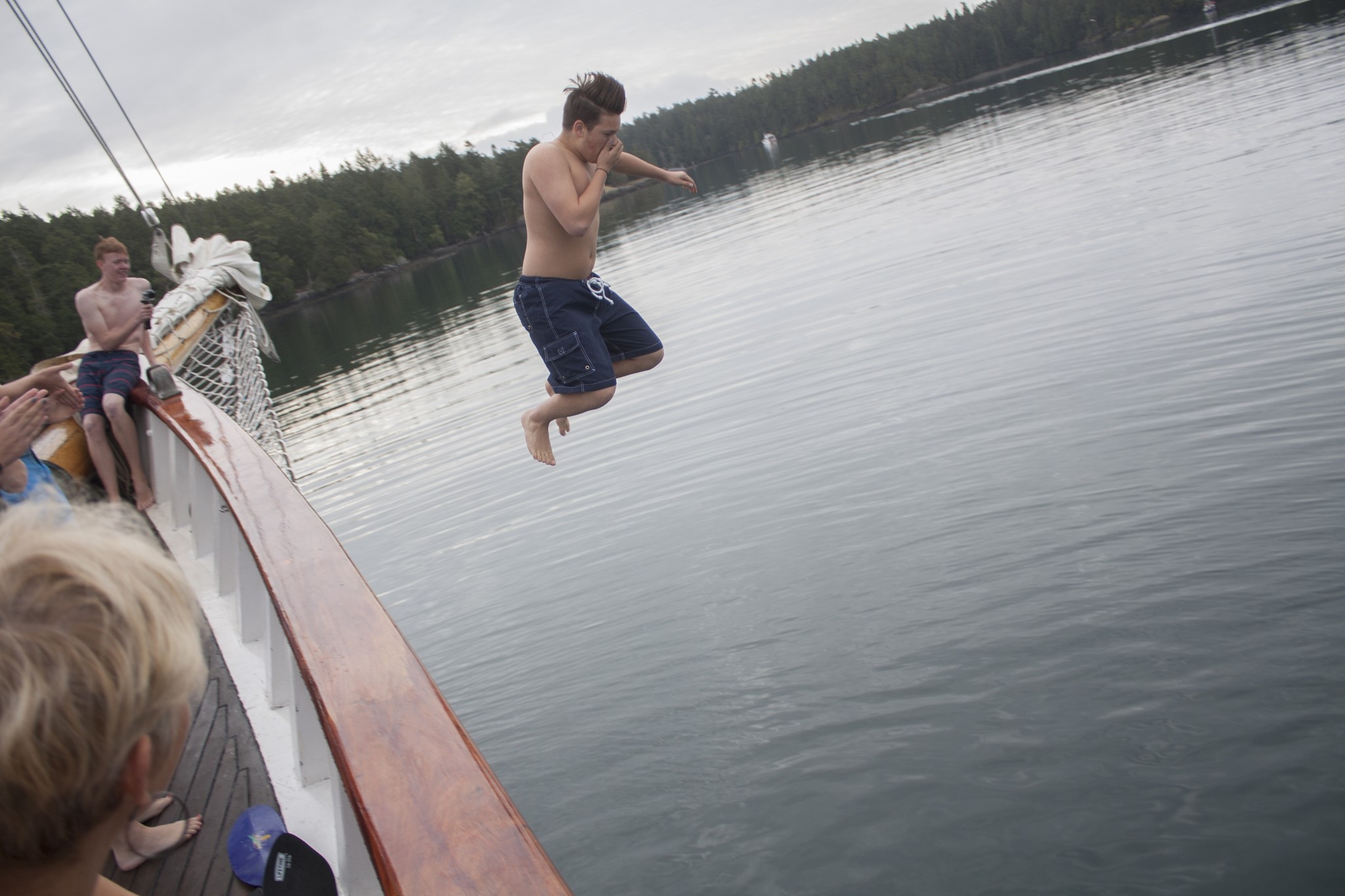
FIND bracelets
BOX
[595,168,608,176]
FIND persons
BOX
[0,498,207,896]
[75,237,171,510]
[512,72,697,467]
[0,363,203,870]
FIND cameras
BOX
[141,287,156,303]
